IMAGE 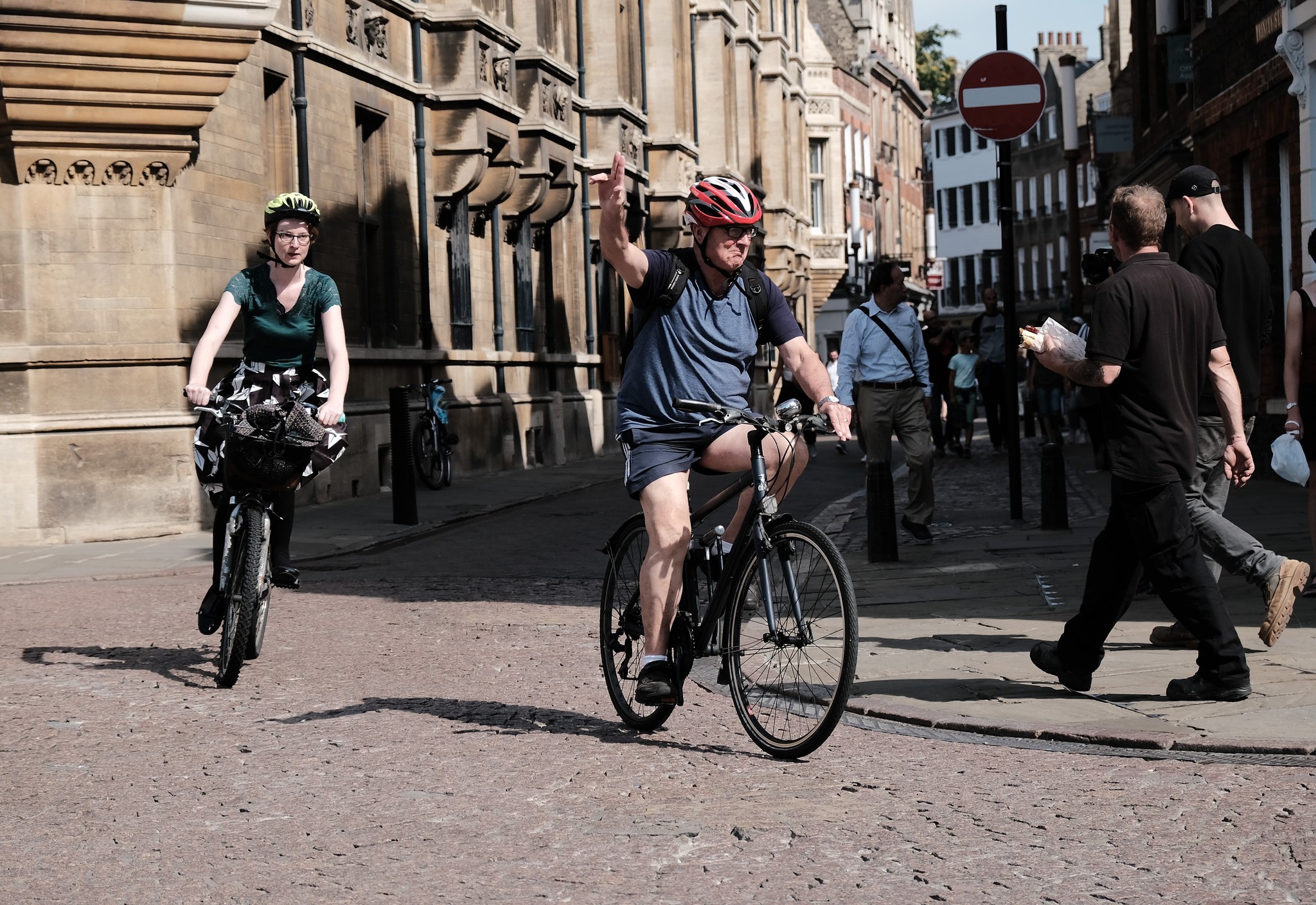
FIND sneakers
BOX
[1257,557,1311,648]
[698,543,759,611]
[634,659,675,704]
[1149,620,1201,650]
[901,516,933,544]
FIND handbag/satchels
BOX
[230,400,326,486]
[1271,433,1310,487]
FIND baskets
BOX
[223,426,316,499]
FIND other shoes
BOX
[957,448,962,456]
[836,440,849,455]
[945,434,962,450]
[1030,642,1093,692]
[939,446,945,454]
[995,446,1001,455]
[196,585,229,635]
[1166,672,1253,701]
[808,444,817,459]
[963,448,972,457]
[861,454,867,462]
[271,566,300,585]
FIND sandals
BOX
[1302,572,1316,596]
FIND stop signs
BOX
[955,49,1048,143]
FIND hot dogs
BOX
[1019,325,1039,349]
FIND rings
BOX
[843,420,848,421]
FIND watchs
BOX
[816,396,840,409]
[1285,402,1298,410]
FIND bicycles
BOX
[594,398,861,761]
[404,378,460,491]
[182,387,338,689]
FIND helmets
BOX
[683,176,762,226]
[264,192,321,227]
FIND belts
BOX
[862,376,928,391]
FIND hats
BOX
[1164,165,1230,209]
[959,330,977,341]
[925,322,942,338]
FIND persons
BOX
[185,193,352,636]
[590,152,853,702]
[768,164,1316,702]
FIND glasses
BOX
[275,230,313,244]
[715,224,759,238]
